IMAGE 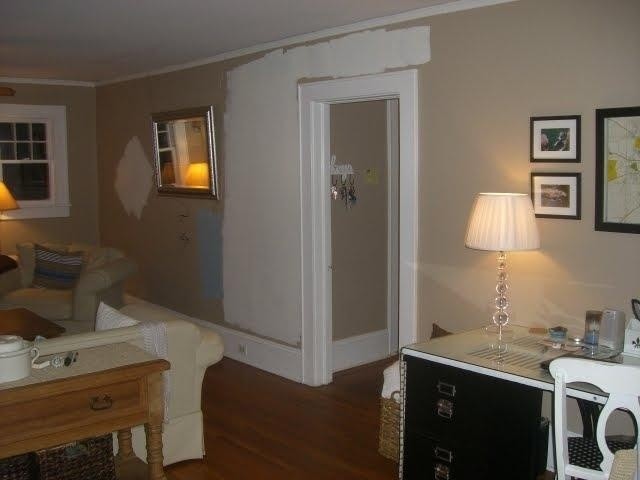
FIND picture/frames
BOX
[594,106,640,234]
[529,115,581,163]
[531,172,581,220]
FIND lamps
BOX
[0,180,19,211]
[464,192,541,343]
[184,162,210,187]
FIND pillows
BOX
[31,242,89,289]
[94,302,142,332]
[1,307,66,341]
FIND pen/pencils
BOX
[64,351,78,366]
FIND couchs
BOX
[0,298,225,468]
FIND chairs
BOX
[1,239,129,320]
[548,357,640,480]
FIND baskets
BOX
[378,391,400,462]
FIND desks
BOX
[399,324,640,480]
[1,342,171,480]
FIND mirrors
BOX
[149,104,220,200]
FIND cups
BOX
[583,310,604,347]
